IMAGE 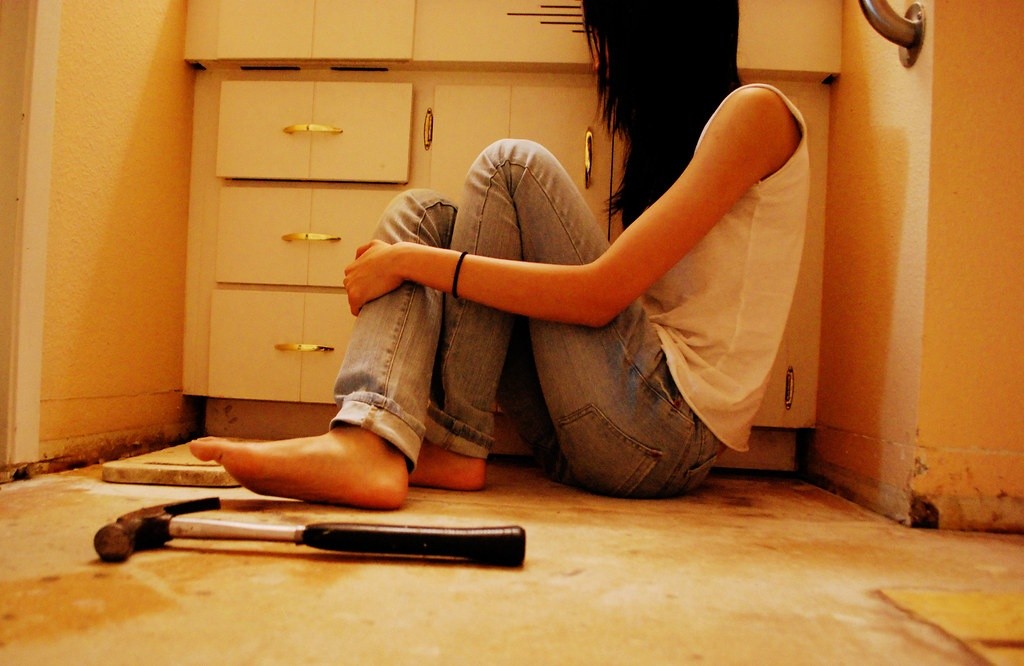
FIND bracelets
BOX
[450,249,469,299]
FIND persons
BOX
[186,2,812,511]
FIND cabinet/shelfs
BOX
[182,0,844,475]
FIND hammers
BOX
[92,496,527,564]
